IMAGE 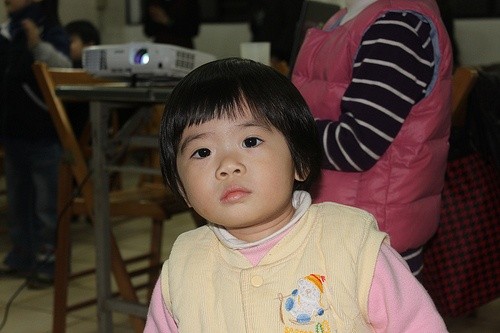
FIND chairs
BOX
[33,61,206,333]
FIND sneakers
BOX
[0,243,37,273]
[26,245,72,289]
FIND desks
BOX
[53,85,179,333]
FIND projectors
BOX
[82,42,217,78]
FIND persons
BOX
[290,0,453,278]
[143,57,449,333]
[0,0,303,288]
